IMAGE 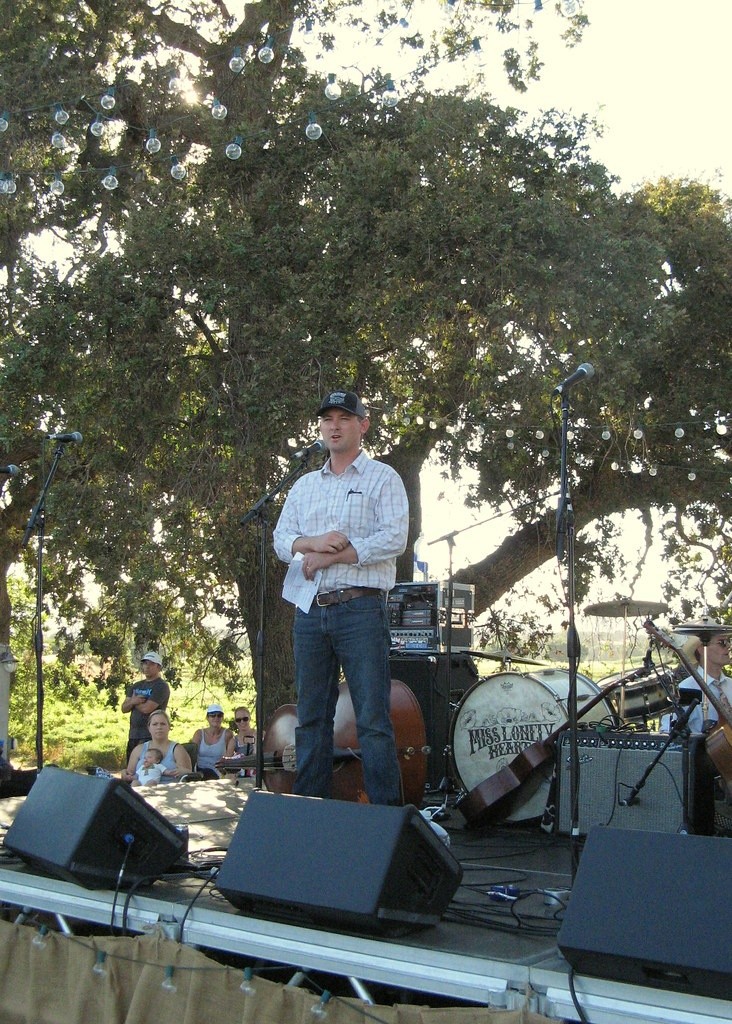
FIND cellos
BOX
[214,678,431,809]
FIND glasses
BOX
[235,717,248,723]
[209,713,223,717]
[710,639,730,647]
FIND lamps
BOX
[558,827,732,1002]
[3,767,190,891]
[215,788,463,939]
[0,643,20,674]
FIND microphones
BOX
[620,797,640,807]
[289,439,325,460]
[0,464,19,476]
[565,487,575,521]
[643,641,653,667]
[45,432,83,443]
[551,363,594,396]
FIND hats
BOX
[315,390,365,418]
[684,615,732,666]
[207,705,223,712]
[141,652,162,665]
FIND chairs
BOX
[178,734,264,782]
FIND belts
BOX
[312,587,384,604]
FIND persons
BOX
[122,652,170,768]
[129,748,169,787]
[660,634,732,734]
[224,707,257,777]
[121,709,192,787]
[273,391,409,809]
[191,704,232,782]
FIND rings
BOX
[307,566,309,569]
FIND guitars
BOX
[642,619,732,793]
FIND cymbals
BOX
[671,615,732,637]
[584,598,669,618]
[460,648,548,666]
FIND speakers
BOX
[3,767,186,889]
[215,791,464,939]
[557,826,732,1001]
[391,652,479,794]
[556,730,714,837]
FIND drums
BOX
[446,666,617,827]
[595,666,679,723]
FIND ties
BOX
[711,681,732,716]
